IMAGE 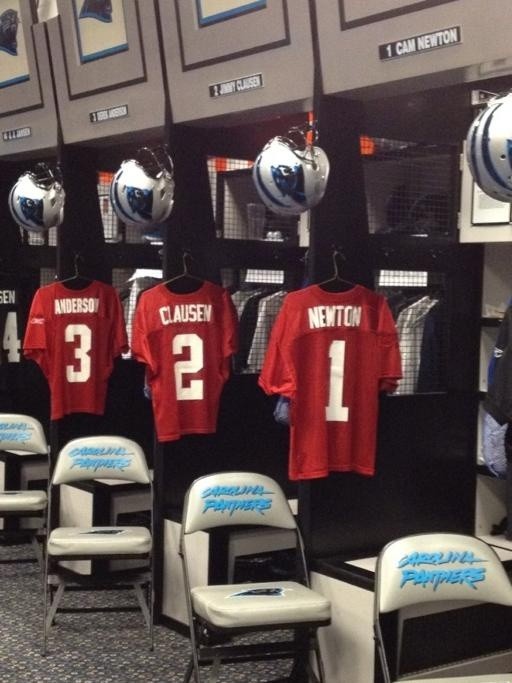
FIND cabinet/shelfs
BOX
[49,469,159,583]
[156,497,304,639]
[0,444,53,529]
[300,534,511,682]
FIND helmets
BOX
[465,90,512,202]
[112,145,175,228]
[254,122,330,216]
[7,157,66,229]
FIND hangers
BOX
[164,253,205,285]
[62,254,91,283]
[319,251,353,288]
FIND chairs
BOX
[0,412,51,562]
[179,470,334,683]
[372,530,511,683]
[39,434,156,658]
